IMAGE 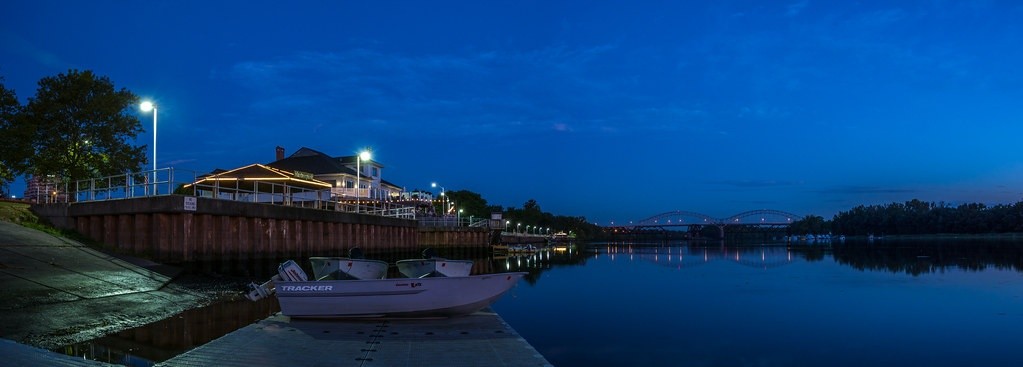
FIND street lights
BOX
[470,216,474,225]
[356,152,368,213]
[458,210,462,226]
[526,225,530,233]
[533,226,536,234]
[517,224,520,233]
[141,101,157,196]
[547,228,549,234]
[506,221,509,232]
[433,184,444,216]
[539,228,542,234]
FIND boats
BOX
[508,244,536,253]
[239,257,529,319]
